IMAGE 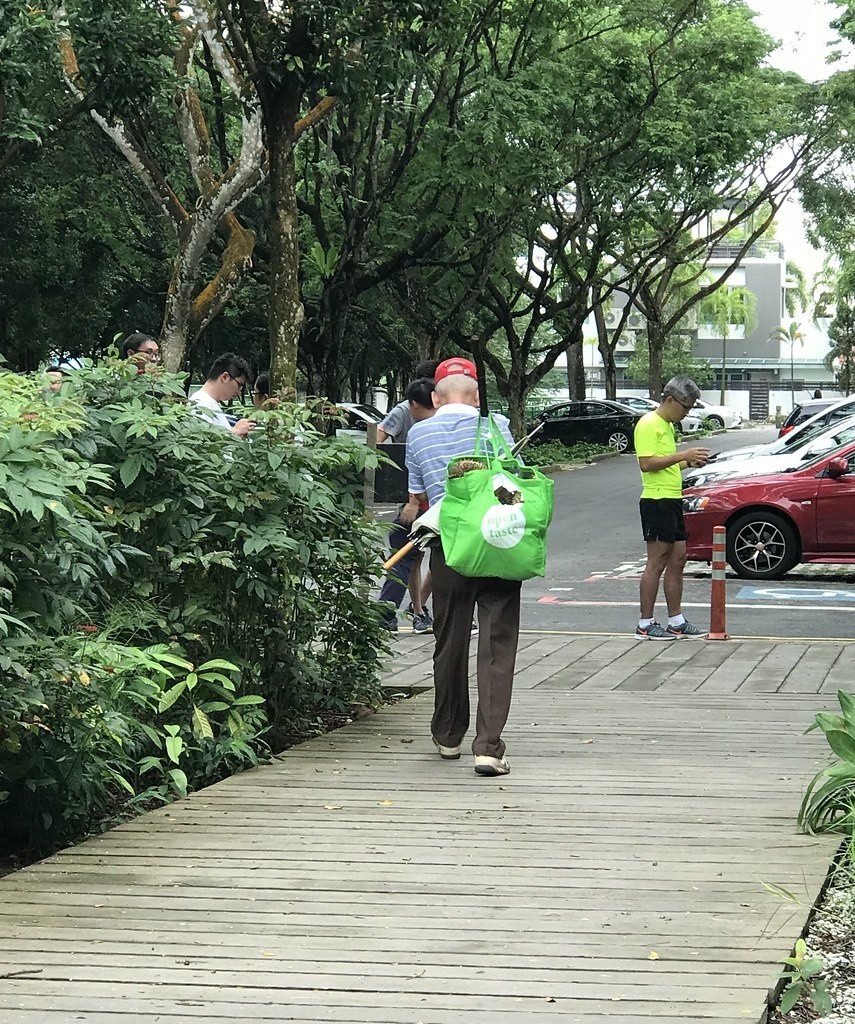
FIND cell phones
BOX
[707,452,721,460]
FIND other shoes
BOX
[431,732,461,759]
[474,753,510,775]
[377,617,399,635]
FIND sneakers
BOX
[470,617,479,635]
[635,621,676,641]
[666,619,709,639]
[412,613,433,634]
[404,601,433,625]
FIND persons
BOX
[121,333,161,369]
[634,376,709,641]
[188,352,256,463]
[375,360,478,635]
[246,374,312,483]
[404,358,525,775]
[44,366,73,394]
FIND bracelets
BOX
[687,461,692,468]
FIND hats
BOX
[434,357,478,385]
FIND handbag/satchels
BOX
[438,410,554,581]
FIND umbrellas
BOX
[383,422,545,571]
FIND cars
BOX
[684,398,742,432]
[223,402,394,444]
[680,437,855,580]
[525,397,646,454]
[600,397,703,435]
[682,412,855,493]
[705,393,855,466]
[777,397,846,440]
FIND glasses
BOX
[221,370,244,391]
[132,348,161,362]
[251,390,269,398]
[667,393,693,412]
